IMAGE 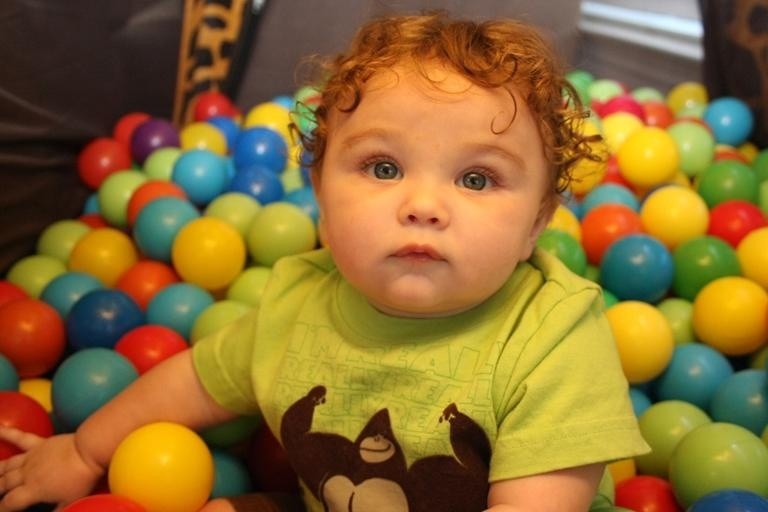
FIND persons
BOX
[0,9,652,512]
